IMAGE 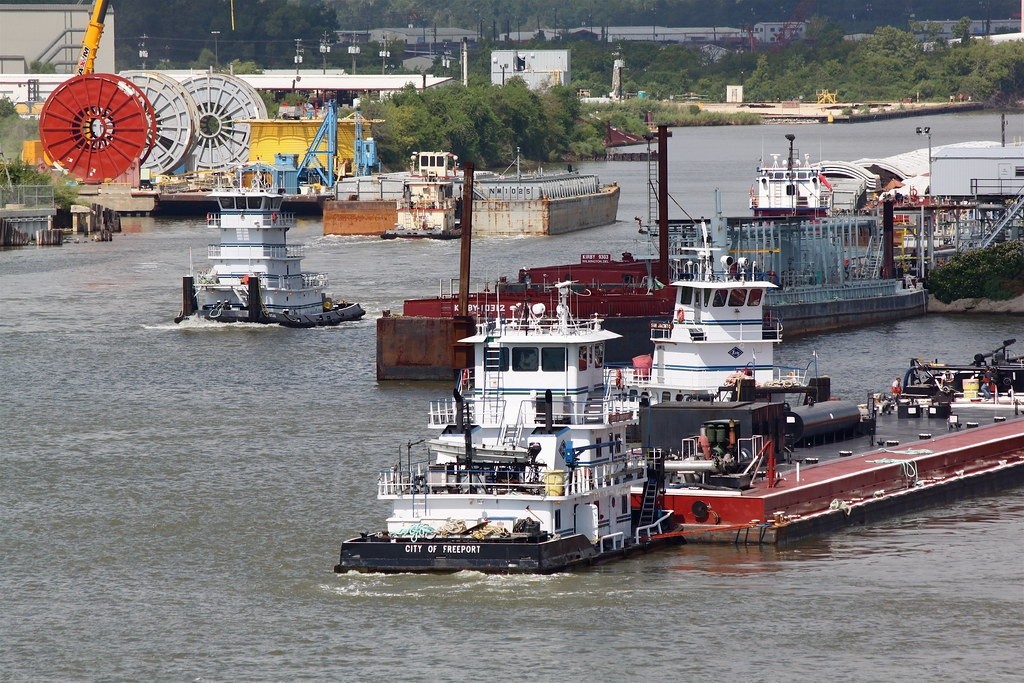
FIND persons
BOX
[910,248,917,268]
[980,365,992,401]
[891,377,902,404]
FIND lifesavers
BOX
[614,368,624,391]
[244,275,249,283]
[272,211,279,223]
[206,213,213,223]
[674,306,686,325]
[460,367,471,386]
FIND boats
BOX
[174,163,367,328]
[380,148,463,240]
[333,276,1024,575]
[749,133,831,218]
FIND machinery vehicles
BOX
[15,0,109,168]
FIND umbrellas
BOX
[885,178,906,195]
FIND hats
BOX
[985,366,990,368]
[896,376,901,380]
[382,530,389,533]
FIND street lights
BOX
[347,31,361,76]
[137,32,149,70]
[441,41,451,78]
[914,127,932,201]
[292,37,304,77]
[377,32,391,77]
[319,29,332,75]
